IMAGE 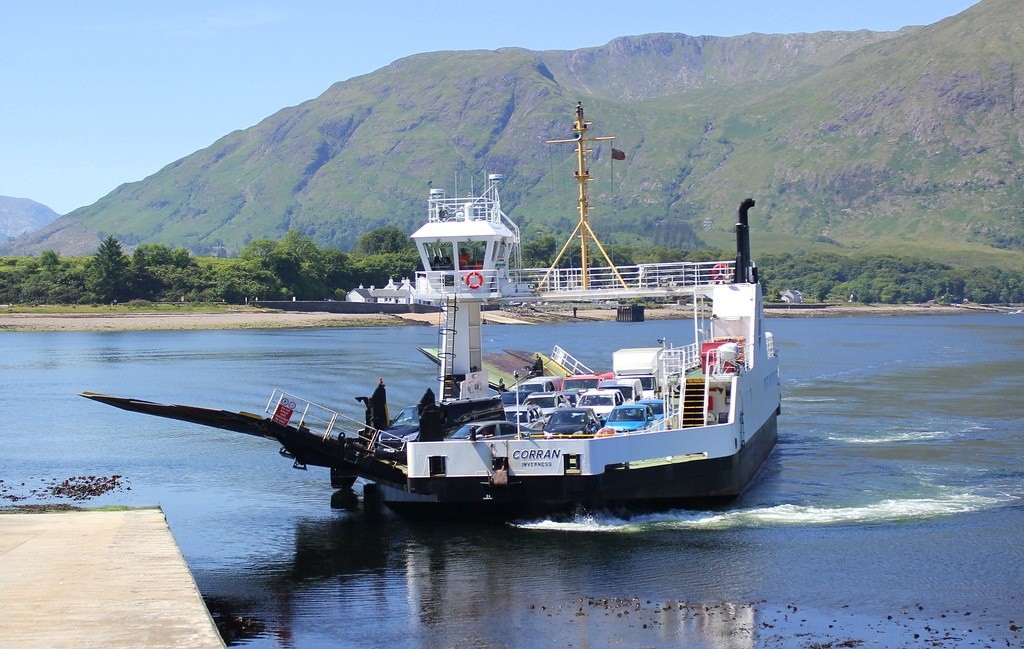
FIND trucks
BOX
[613,348,663,400]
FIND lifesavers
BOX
[465,271,484,290]
[711,263,730,281]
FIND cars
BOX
[379,406,420,454]
[443,372,673,441]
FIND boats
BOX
[78,102,782,508]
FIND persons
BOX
[459,249,470,270]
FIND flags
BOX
[612,149,625,160]
